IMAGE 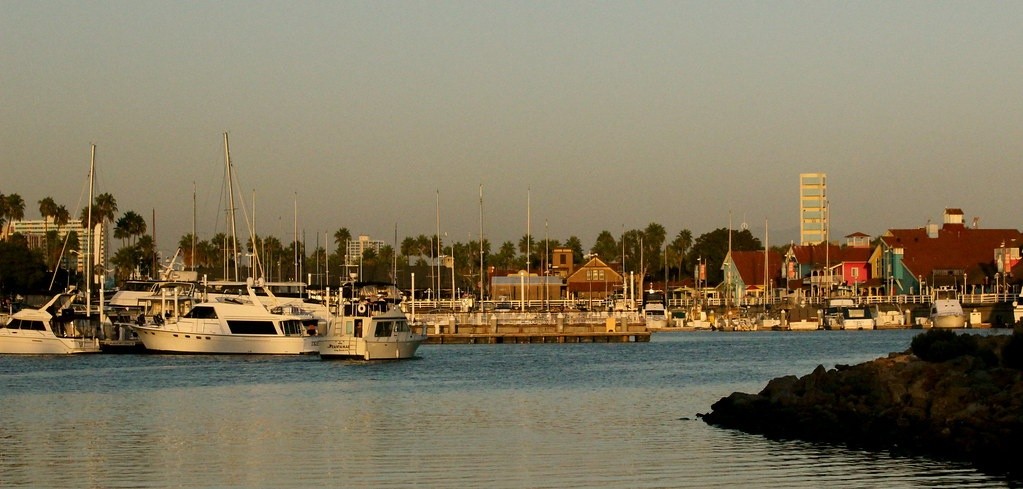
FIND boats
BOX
[324,237,431,362]
[828,298,874,330]
[872,301,905,328]
[926,272,968,330]
[644,302,669,330]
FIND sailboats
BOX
[0,132,142,353]
[130,130,320,355]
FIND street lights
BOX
[444,231,457,310]
[697,254,701,303]
[588,251,599,312]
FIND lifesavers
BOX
[358,304,366,313]
